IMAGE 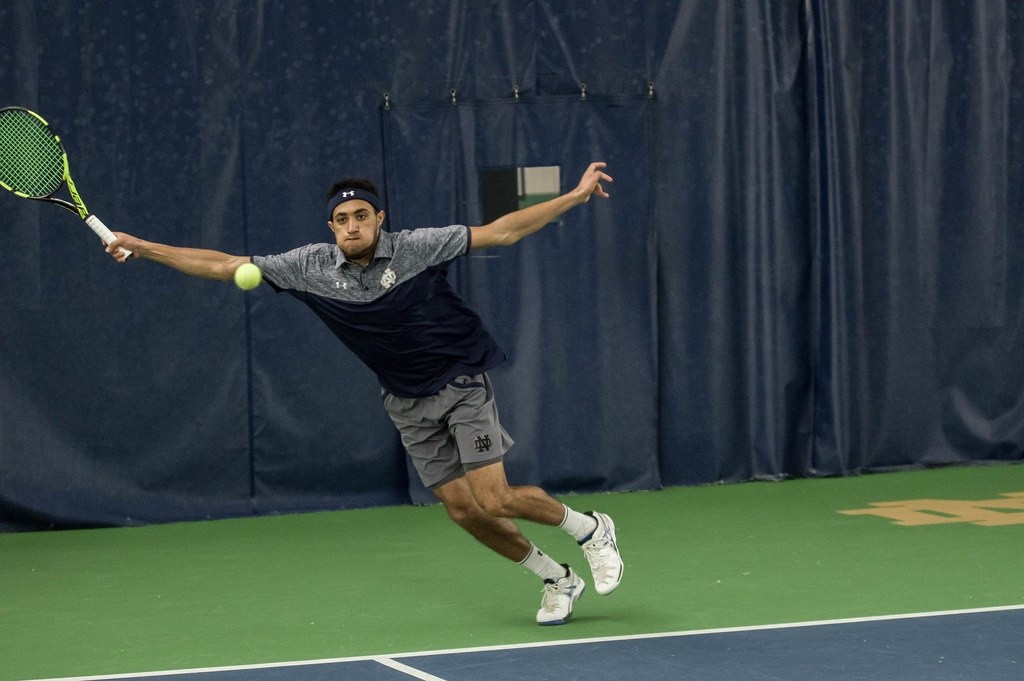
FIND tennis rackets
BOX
[0,106,131,261]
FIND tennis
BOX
[234,263,261,290]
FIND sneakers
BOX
[536,562,585,625]
[576,510,624,595]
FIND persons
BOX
[97,160,624,626]
